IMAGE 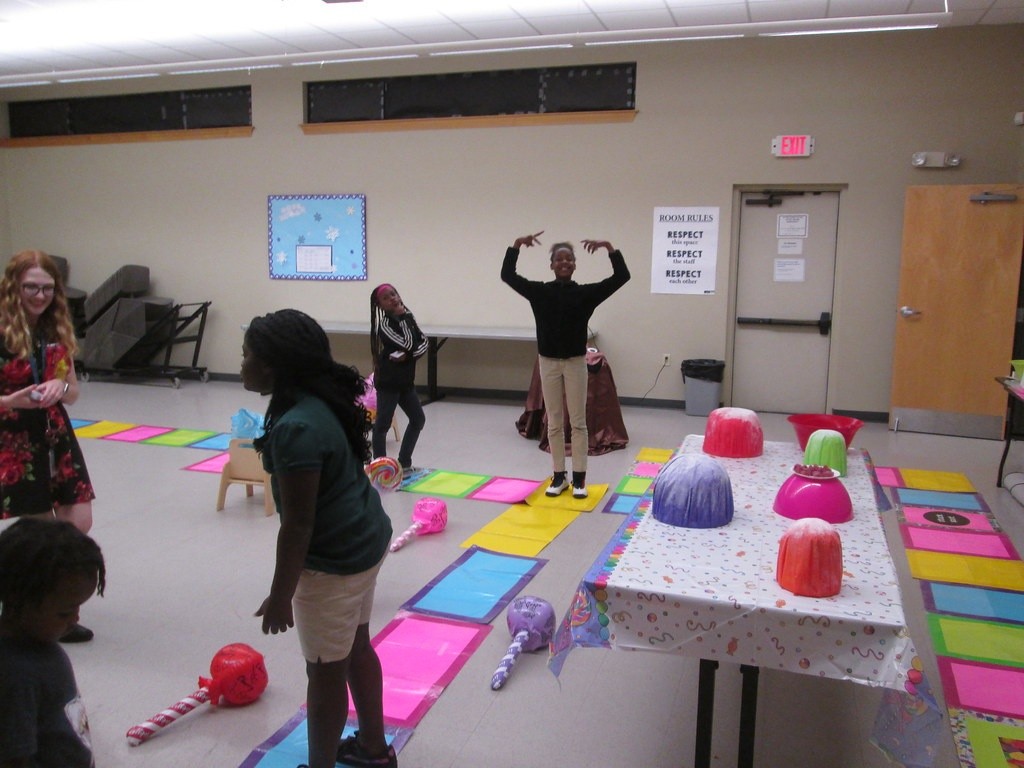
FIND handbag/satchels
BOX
[229,408,270,449]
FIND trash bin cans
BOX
[682,359,725,417]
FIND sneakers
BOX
[401,465,423,478]
[335,731,397,768]
[545,470,569,497]
[571,471,587,499]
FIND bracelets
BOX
[64,382,69,394]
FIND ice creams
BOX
[356,372,377,424]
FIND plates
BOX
[790,462,842,479]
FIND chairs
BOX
[216,438,274,517]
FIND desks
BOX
[240,318,600,405]
[995,375,1024,488]
[546,434,945,768]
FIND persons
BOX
[371,284,429,470]
[501,230,631,498]
[0,518,106,768]
[0,253,95,642]
[241,310,398,768]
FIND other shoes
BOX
[57,622,94,643]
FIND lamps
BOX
[912,151,963,168]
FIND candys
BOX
[364,457,403,494]
[491,595,555,686]
[126,643,268,746]
[390,497,448,549]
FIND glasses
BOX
[17,282,59,298]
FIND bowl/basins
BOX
[773,465,853,524]
[787,414,864,452]
[1009,360,1024,379]
